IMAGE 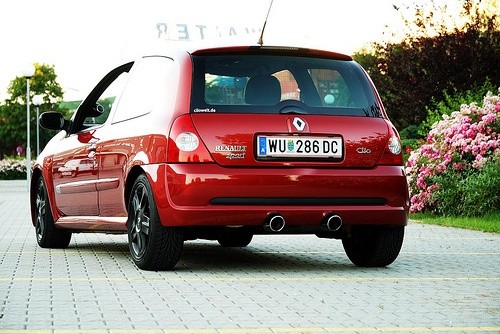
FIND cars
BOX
[30,38,412,271]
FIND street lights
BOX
[31,95,43,157]
[22,63,36,193]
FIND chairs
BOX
[244,73,282,105]
